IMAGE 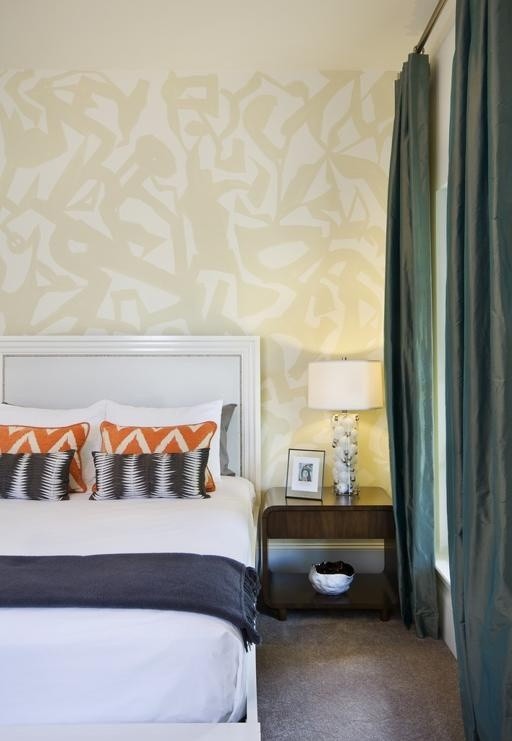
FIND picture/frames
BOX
[286,447,326,503]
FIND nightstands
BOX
[257,484,400,622]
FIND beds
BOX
[1,333,267,741]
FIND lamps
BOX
[303,355,383,497]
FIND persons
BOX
[300,465,311,481]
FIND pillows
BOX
[2,399,240,504]
